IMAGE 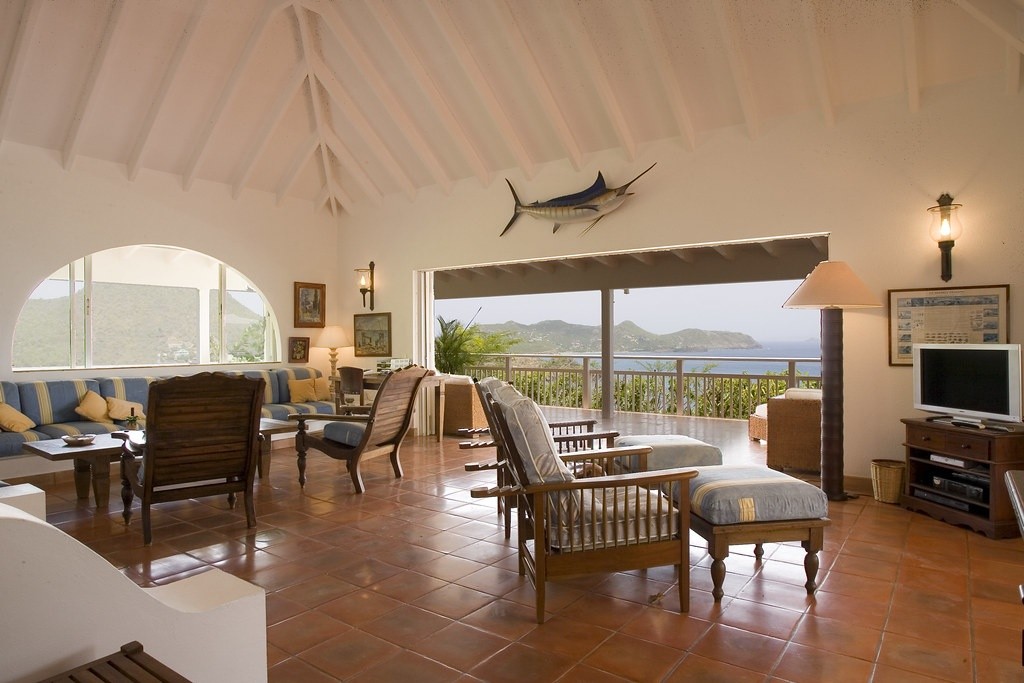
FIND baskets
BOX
[871,459,906,503]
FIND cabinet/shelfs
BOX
[897,415,1024,540]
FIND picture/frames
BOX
[886,284,1011,367]
[293,281,326,328]
[353,312,392,358]
[288,336,310,364]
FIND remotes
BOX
[951,419,986,431]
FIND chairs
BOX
[463,385,700,625]
[456,375,621,542]
[0,381,51,458]
[19,378,127,439]
[284,364,429,496]
[275,366,337,415]
[38,641,193,683]
[100,376,166,430]
[767,393,821,477]
[434,375,489,438]
[117,370,266,548]
[336,366,364,406]
[221,370,304,422]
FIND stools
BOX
[613,433,723,491]
[747,413,767,442]
[661,462,832,604]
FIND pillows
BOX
[0,402,37,433]
[105,396,146,421]
[74,390,114,425]
[287,378,317,403]
[314,377,333,401]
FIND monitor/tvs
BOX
[913,343,1023,432]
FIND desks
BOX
[21,417,309,509]
[328,374,450,442]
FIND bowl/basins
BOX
[61,434,96,445]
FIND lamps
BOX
[353,261,375,311]
[314,324,353,394]
[926,192,963,283]
[779,259,885,503]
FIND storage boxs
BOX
[929,453,975,469]
[376,361,391,376]
[391,359,409,371]
[785,388,822,400]
